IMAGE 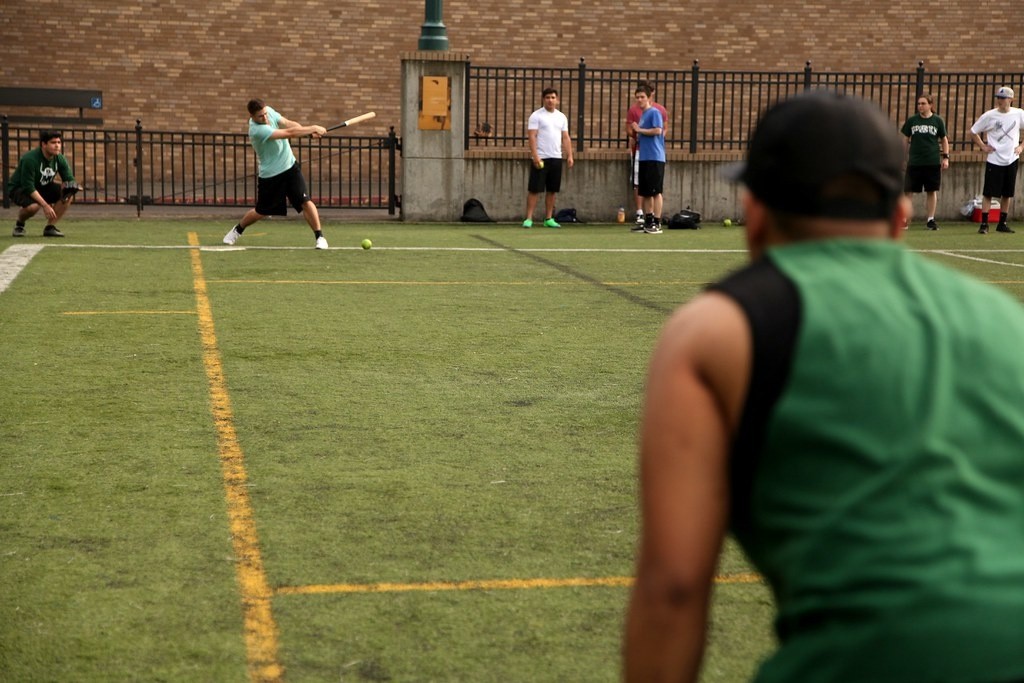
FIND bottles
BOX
[617,204,625,223]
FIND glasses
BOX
[997,96,1007,99]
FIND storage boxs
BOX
[970,200,1001,222]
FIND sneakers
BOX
[978,222,989,233]
[522,218,533,228]
[996,224,1015,233]
[223,226,241,245]
[315,236,328,249]
[13,223,25,237]
[635,213,645,224]
[927,219,940,230]
[543,218,561,227]
[899,221,908,229]
[644,223,663,233]
[631,223,656,232]
[44,225,64,236]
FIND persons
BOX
[970,86,1024,234]
[9,129,79,238]
[901,94,950,231]
[626,81,668,235]
[223,99,330,251]
[522,88,574,228]
[616,90,1022,683]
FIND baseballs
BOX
[724,219,732,226]
[538,161,544,169]
[361,239,372,250]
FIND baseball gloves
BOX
[60,181,84,205]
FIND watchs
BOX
[943,154,950,158]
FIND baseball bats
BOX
[310,110,377,138]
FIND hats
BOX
[721,92,905,218]
[993,87,1014,98]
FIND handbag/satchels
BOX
[557,208,583,223]
[668,210,701,229]
